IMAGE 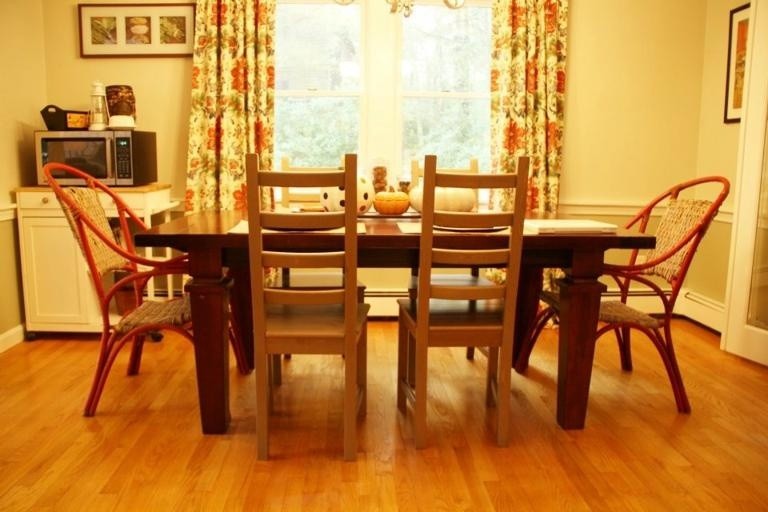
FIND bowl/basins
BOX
[373,200,410,215]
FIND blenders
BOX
[88,81,109,131]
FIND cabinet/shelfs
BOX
[15,187,181,341]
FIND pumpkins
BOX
[409,184,476,213]
[373,185,409,215]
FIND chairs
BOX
[249,154,369,459]
[515,177,731,414]
[395,155,530,449]
[43,162,250,416]
[408,157,479,360]
[273,158,366,387]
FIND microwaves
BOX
[33,130,158,186]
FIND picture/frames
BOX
[722,1,751,123]
[78,2,196,59]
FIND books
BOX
[508,218,620,235]
